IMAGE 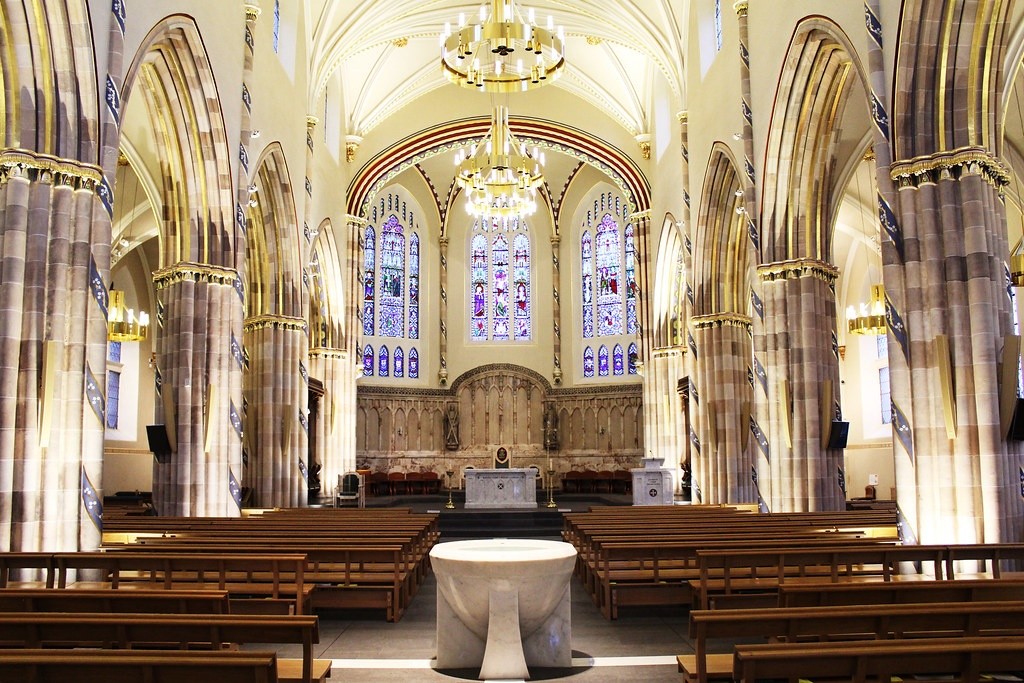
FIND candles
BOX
[550,459,552,470]
[449,459,452,472]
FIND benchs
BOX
[554,500,1024,682]
[0,506,441,683]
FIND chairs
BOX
[371,472,442,495]
[330,471,366,509]
[562,470,631,494]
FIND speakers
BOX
[1007,398,1024,441]
[146,424,170,453]
[827,421,849,449]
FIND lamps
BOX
[845,144,888,336]
[437,1,567,94]
[453,105,548,224]
[108,162,150,345]
